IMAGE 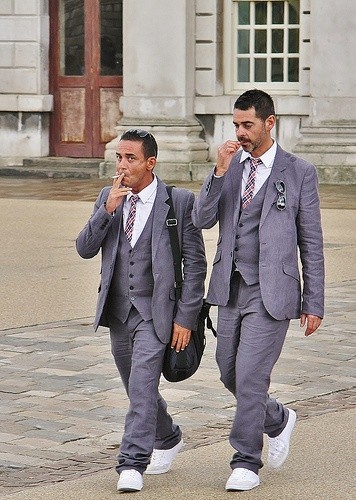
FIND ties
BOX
[242,158,263,209]
[124,195,140,242]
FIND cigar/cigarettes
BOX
[112,175,120,179]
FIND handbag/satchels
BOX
[162,299,217,382]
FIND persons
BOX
[191,90,325,491]
[76,130,207,491]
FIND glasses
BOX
[272,180,287,211]
[123,128,151,139]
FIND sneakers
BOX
[225,468,259,491]
[266,408,296,468]
[144,440,183,474]
[116,469,143,491]
